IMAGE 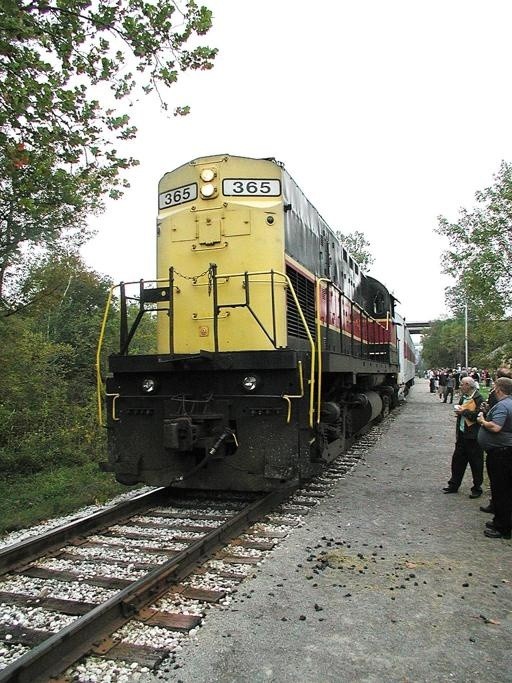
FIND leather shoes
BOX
[480,506,496,514]
[469,494,481,499]
[443,487,458,493]
[484,519,511,539]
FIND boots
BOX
[441,401,453,404]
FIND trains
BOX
[96,154,415,493]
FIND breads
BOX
[478,412,483,416]
[454,405,460,410]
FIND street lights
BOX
[459,302,468,369]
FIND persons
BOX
[424,364,512,540]
